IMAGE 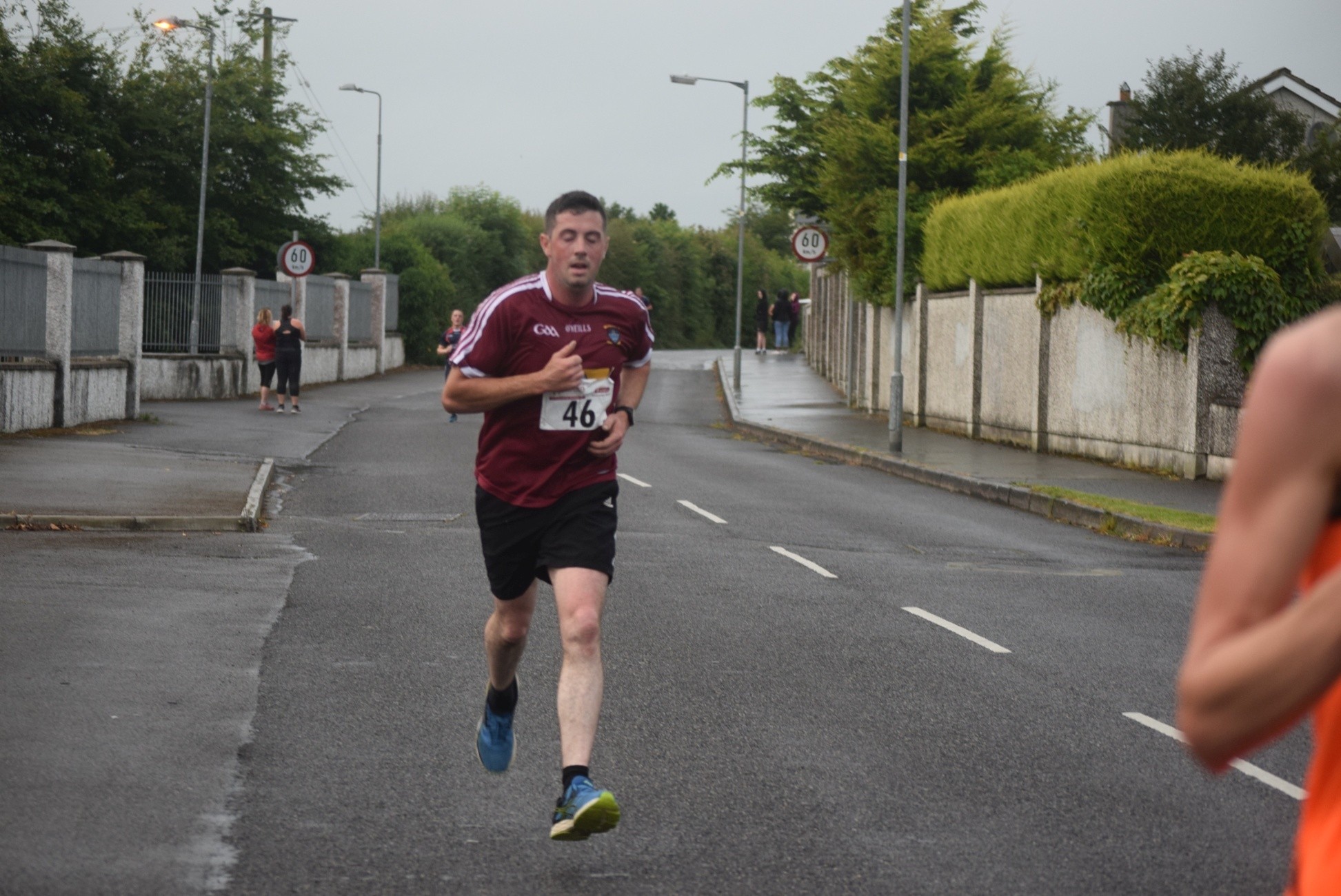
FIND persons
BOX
[789,292,799,347]
[436,309,467,423]
[754,290,768,356]
[632,287,654,311]
[443,190,659,841]
[273,304,305,415]
[772,290,792,351]
[1175,294,1341,896]
[253,308,276,411]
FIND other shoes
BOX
[762,350,766,354]
[290,405,301,414]
[755,351,760,354]
[260,403,276,411]
[449,415,457,422]
[277,404,284,413]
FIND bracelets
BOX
[613,405,637,428]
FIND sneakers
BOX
[476,669,521,774]
[550,776,620,841]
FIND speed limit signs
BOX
[792,226,828,263]
[282,241,315,277]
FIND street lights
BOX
[340,85,383,269]
[150,17,215,354]
[669,74,749,399]
